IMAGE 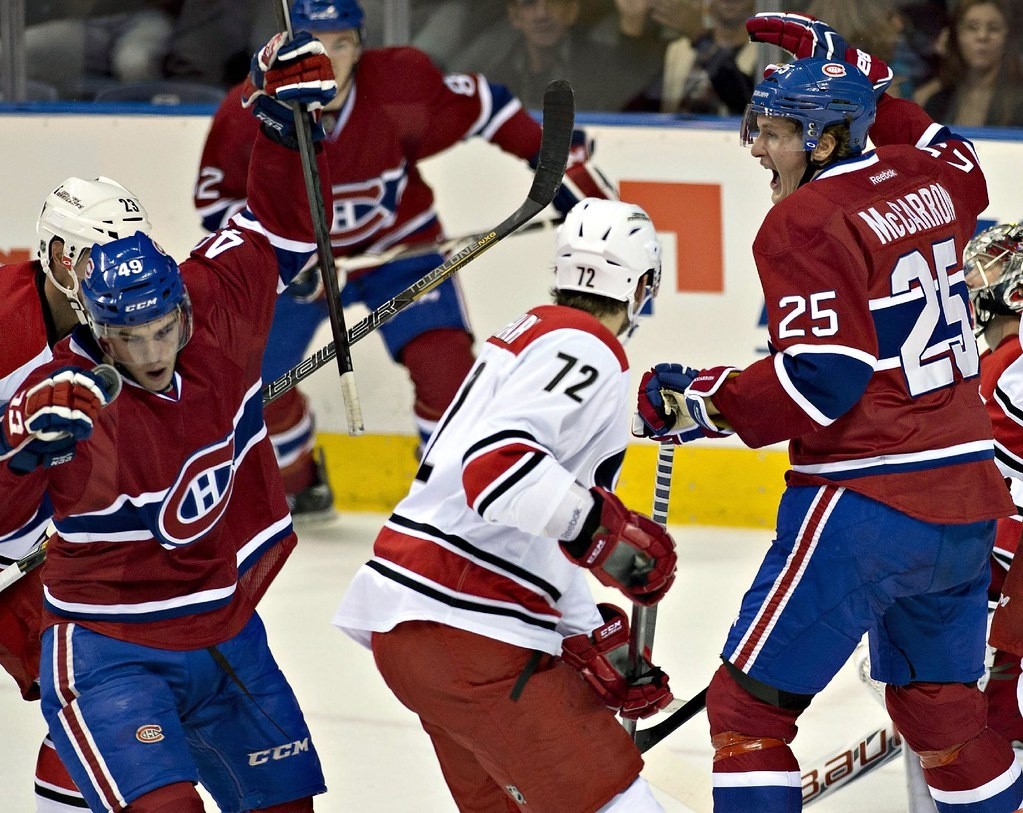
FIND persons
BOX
[193,0,616,516]
[0,175,152,813]
[488,0,1023,127]
[334,198,677,813]
[0,31,338,813]
[962,225,1023,813]
[633,11,1023,813]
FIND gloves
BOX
[529,129,621,214]
[565,603,674,721]
[745,10,892,98]
[253,27,338,113]
[3,366,107,446]
[633,361,740,447]
[560,487,680,607]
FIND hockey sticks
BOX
[638,432,688,712]
[260,78,578,403]
[277,1,365,436]
[619,554,712,755]
[803,722,904,806]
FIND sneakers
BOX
[287,447,341,530]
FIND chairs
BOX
[94,82,227,104]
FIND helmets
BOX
[293,0,364,32]
[36,176,153,294]
[752,55,876,152]
[79,231,184,326]
[550,197,659,301]
[962,223,1023,337]
